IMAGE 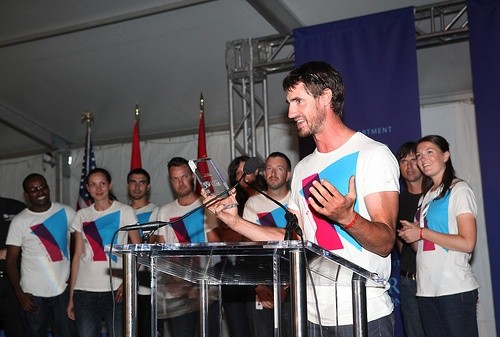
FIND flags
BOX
[131,121,142,170]
[196,112,215,197]
[76,130,97,212]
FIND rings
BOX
[120,293,122,297]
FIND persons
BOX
[199,61,402,337]
[4,135,482,337]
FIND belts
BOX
[399,269,416,280]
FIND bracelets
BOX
[420,227,423,240]
[344,212,359,230]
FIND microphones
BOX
[121,156,304,236]
